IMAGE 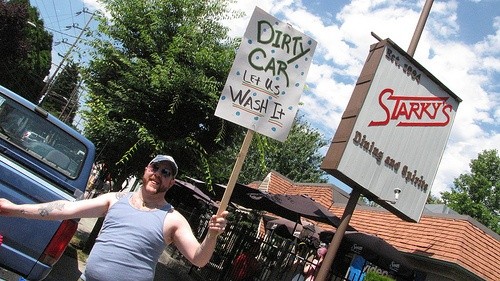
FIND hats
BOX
[298,242,307,248]
[148,155,178,178]
[317,247,327,256]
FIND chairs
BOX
[28,141,70,169]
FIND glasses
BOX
[149,163,172,178]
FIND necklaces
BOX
[139,191,165,208]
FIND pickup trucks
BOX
[0,84,96,281]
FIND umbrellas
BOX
[261,218,323,248]
[342,230,410,276]
[249,188,357,234]
[220,176,266,215]
[175,178,217,207]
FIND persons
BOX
[230,236,328,281]
[0,155,230,281]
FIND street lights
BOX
[61,94,84,123]
[54,52,84,119]
[28,20,74,102]
